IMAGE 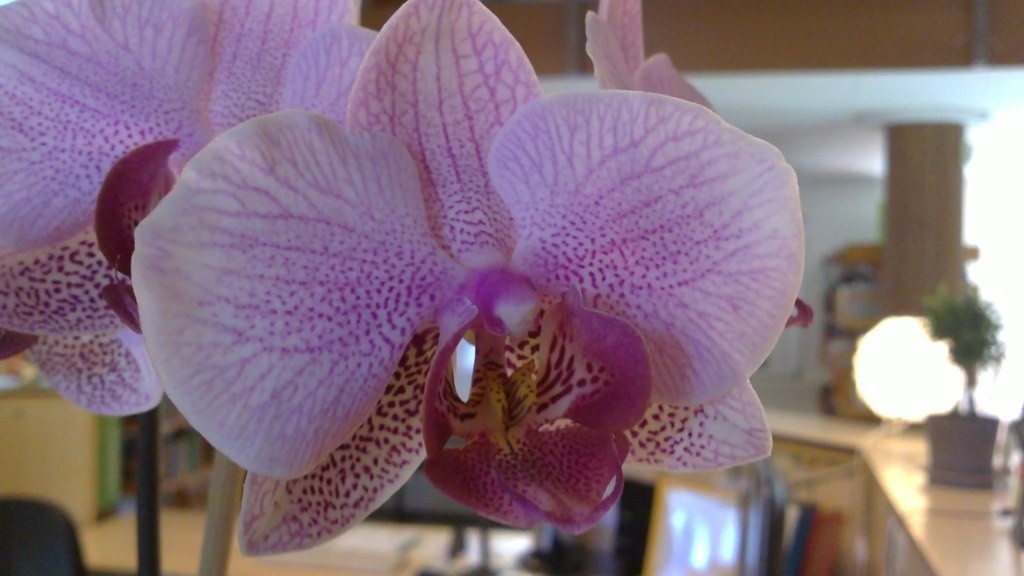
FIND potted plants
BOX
[915,278,1006,490]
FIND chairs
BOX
[0,494,131,576]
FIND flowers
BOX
[131,0,805,557]
[584,0,815,328]
[0,0,378,417]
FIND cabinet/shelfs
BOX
[362,0,1024,80]
